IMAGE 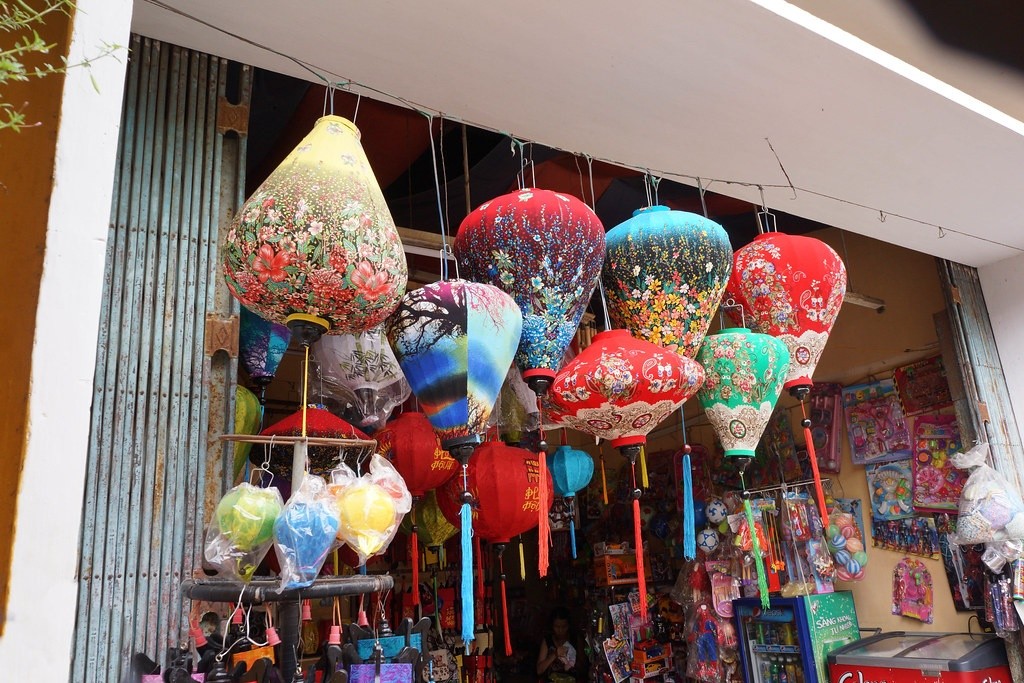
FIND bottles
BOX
[759,653,803,683]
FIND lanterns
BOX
[218,110,851,648]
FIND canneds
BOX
[755,621,799,645]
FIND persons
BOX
[536,605,588,683]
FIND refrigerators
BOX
[828,631,1012,683]
[732,590,860,683]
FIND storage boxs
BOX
[629,642,672,683]
[593,541,653,588]
